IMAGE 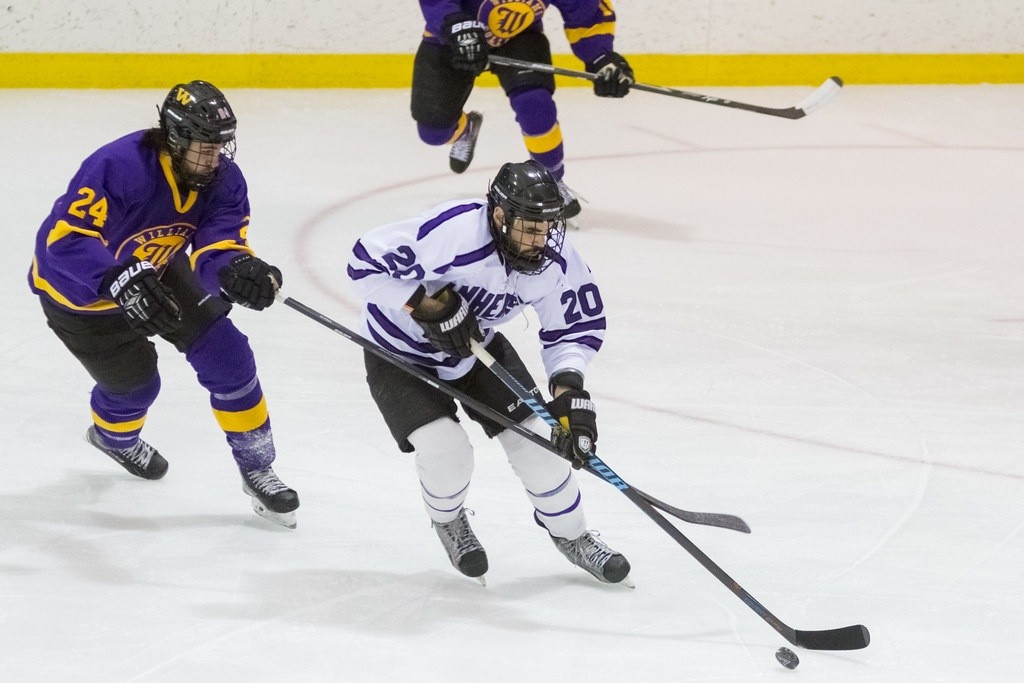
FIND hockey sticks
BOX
[481,54,843,120]
[216,265,751,535]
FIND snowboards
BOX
[464,336,871,650]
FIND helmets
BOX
[486,161,582,270]
[156,80,238,191]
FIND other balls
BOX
[775,647,799,670]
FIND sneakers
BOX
[85,425,169,481]
[556,179,588,230]
[449,110,485,173]
[534,513,635,589]
[431,506,488,588]
[242,465,301,532]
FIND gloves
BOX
[585,51,634,97]
[441,12,487,76]
[218,253,283,312]
[546,390,598,469]
[100,253,185,337]
[411,283,486,358]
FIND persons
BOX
[347,158,645,596]
[409,0,635,230]
[26,78,300,530]
